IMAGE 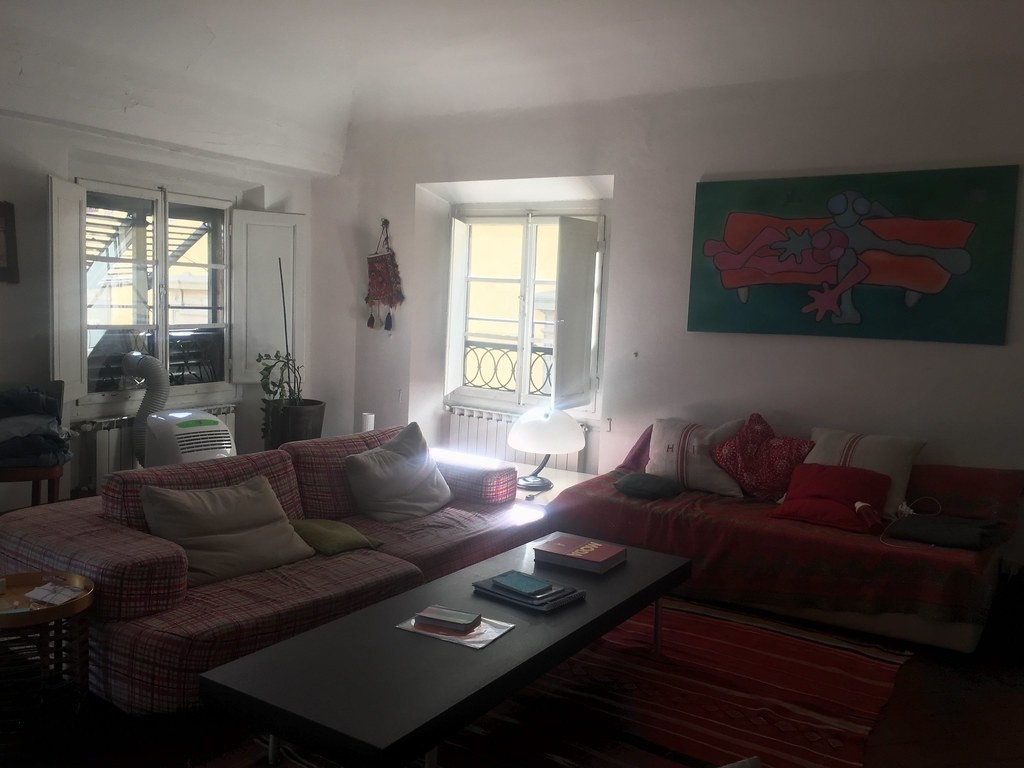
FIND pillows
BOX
[643,416,748,497]
[768,462,889,534]
[290,515,374,558]
[611,470,674,499]
[804,426,927,515]
[343,432,460,524]
[139,474,318,588]
[708,412,815,503]
[0,412,81,458]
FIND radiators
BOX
[83,402,237,493]
[439,403,588,473]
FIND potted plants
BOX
[254,351,325,452]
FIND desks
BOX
[428,442,599,505]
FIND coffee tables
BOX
[198,532,696,768]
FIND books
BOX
[533,535,628,575]
[471,569,586,613]
[413,605,482,635]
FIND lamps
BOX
[508,402,586,493]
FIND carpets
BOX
[190,585,913,768]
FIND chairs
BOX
[0,378,68,505]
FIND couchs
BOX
[551,431,1024,649]
[0,423,552,726]
